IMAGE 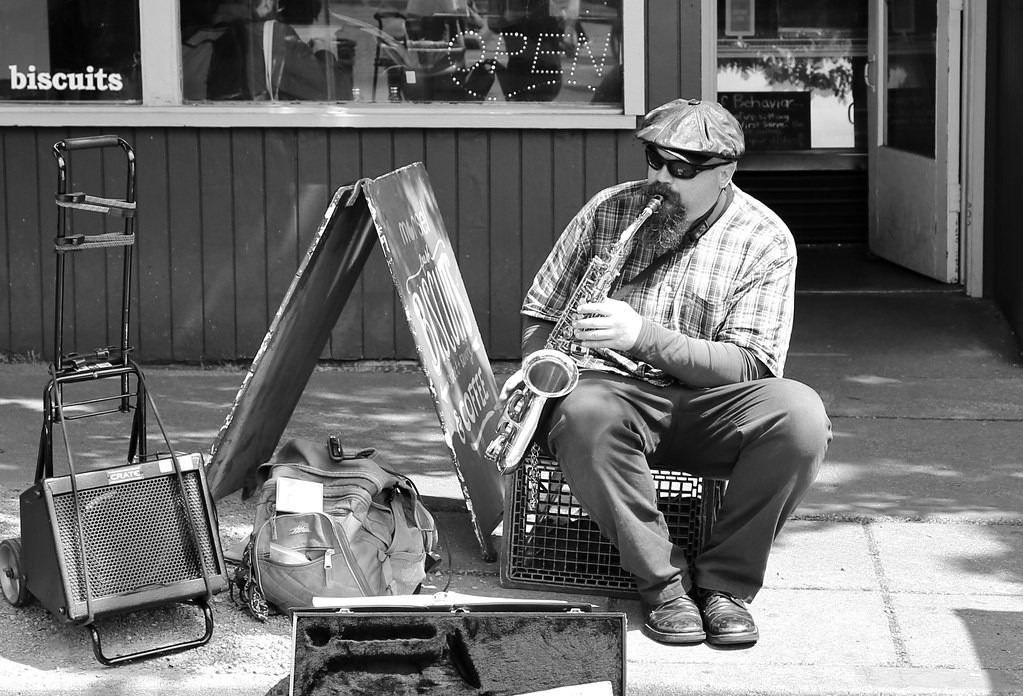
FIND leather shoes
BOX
[643,595,705,643]
[696,586,758,644]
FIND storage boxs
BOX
[499,423,731,595]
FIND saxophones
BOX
[483,195,664,477]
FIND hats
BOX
[634,99,744,159]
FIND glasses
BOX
[645,147,734,179]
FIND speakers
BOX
[19,452,229,624]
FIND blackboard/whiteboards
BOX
[205,161,506,532]
[717,91,812,150]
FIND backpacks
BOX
[235,434,443,622]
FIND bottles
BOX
[350,88,363,100]
[388,87,400,102]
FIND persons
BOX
[180,0,354,102]
[499,98,832,644]
